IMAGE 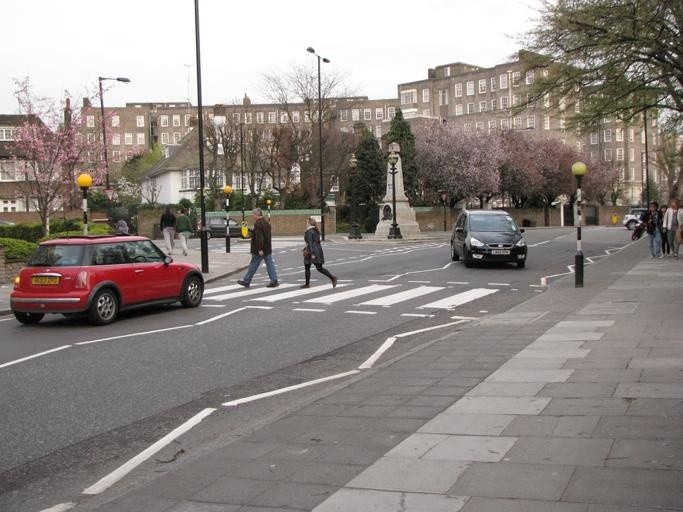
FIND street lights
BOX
[76,174,93,233]
[98,76,137,216]
[194,0,208,272]
[615,70,650,210]
[223,186,232,253]
[498,123,535,204]
[641,149,660,209]
[239,122,245,223]
[267,200,271,224]
[388,147,403,239]
[572,161,587,287]
[307,47,331,241]
[442,119,447,231]
[350,154,362,239]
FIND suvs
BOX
[622,208,647,230]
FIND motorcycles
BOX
[629,221,646,242]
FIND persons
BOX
[160,207,176,254]
[237,208,280,287]
[643,198,683,259]
[300,217,337,288]
[176,208,194,256]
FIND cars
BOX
[0,221,15,227]
[196,216,254,239]
[10,235,205,324]
[450,209,528,269]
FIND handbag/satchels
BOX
[678,227,683,244]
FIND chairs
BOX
[101,248,126,263]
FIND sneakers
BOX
[266,283,279,287]
[237,280,249,287]
[332,277,336,287]
[301,284,309,288]
